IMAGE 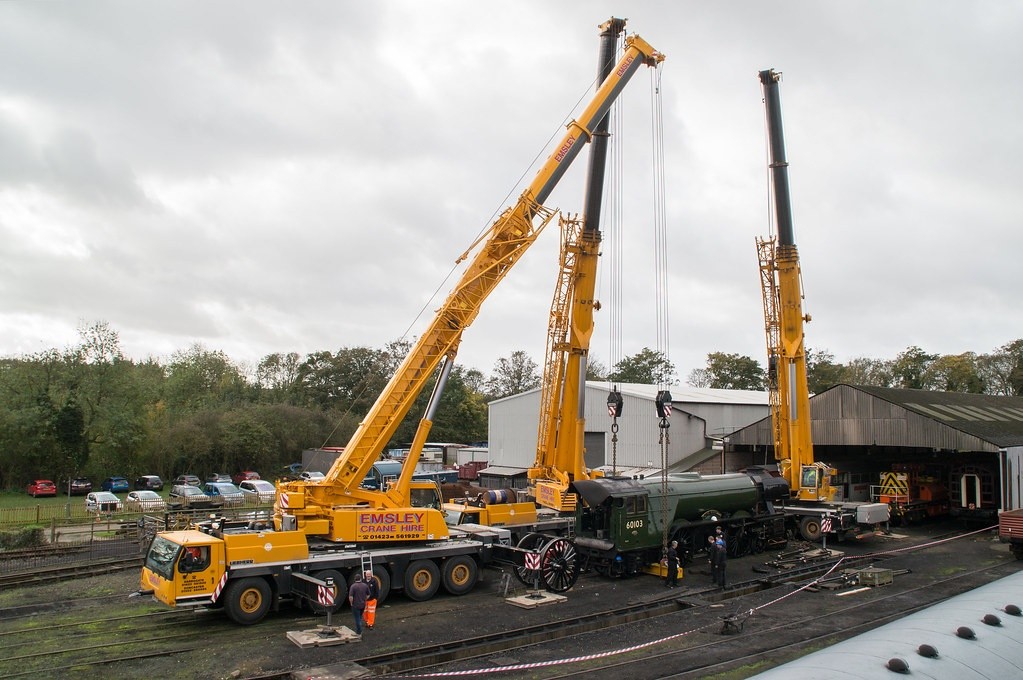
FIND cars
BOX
[100,476,130,494]
[27,480,57,498]
[86,492,124,518]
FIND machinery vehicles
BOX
[134,17,1004,625]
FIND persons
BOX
[716,536,726,547]
[713,539,726,583]
[716,526,726,541]
[668,540,681,589]
[362,569,380,629]
[349,574,370,638]
[708,536,716,575]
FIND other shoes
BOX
[709,580,714,583]
[713,584,719,587]
[674,583,680,587]
[369,625,373,630]
[668,585,674,589]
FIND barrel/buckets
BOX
[483,489,507,504]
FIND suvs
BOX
[62,476,93,495]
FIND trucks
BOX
[997,507,1023,566]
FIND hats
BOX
[716,541,723,545]
